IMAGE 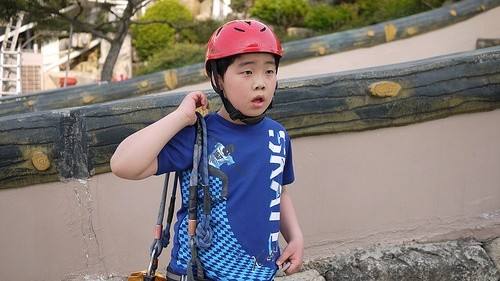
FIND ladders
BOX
[1,45,23,99]
[1,7,25,95]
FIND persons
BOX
[110,19,305,281]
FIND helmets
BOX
[204,19,283,77]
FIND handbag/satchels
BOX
[273,262,325,281]
